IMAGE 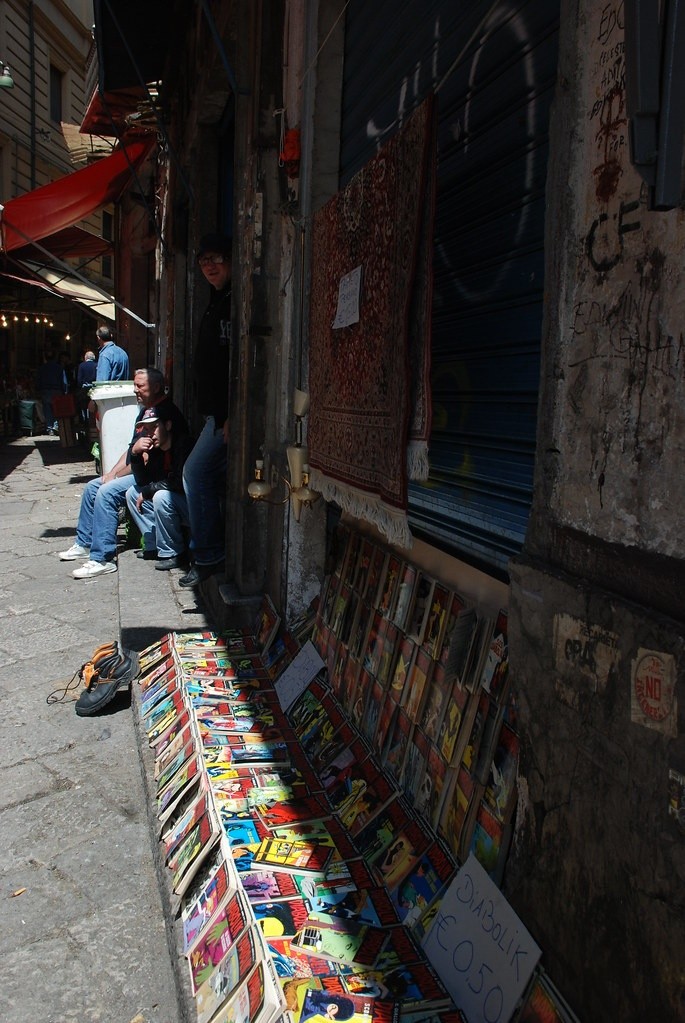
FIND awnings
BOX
[12,225,116,322]
[0,135,155,328]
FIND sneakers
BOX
[58,543,90,560]
[179,559,225,586]
[72,560,117,578]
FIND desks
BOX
[17,398,39,436]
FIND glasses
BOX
[199,255,223,266]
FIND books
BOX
[136,520,578,1023]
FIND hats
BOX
[195,233,231,263]
[135,407,169,427]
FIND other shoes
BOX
[46,641,139,703]
[154,554,189,570]
[137,551,156,558]
[75,655,141,715]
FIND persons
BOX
[76,350,96,422]
[126,405,197,569]
[96,326,130,381]
[36,352,76,435]
[179,232,233,586]
[61,368,192,578]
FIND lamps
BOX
[246,386,324,523]
[0,60,15,89]
